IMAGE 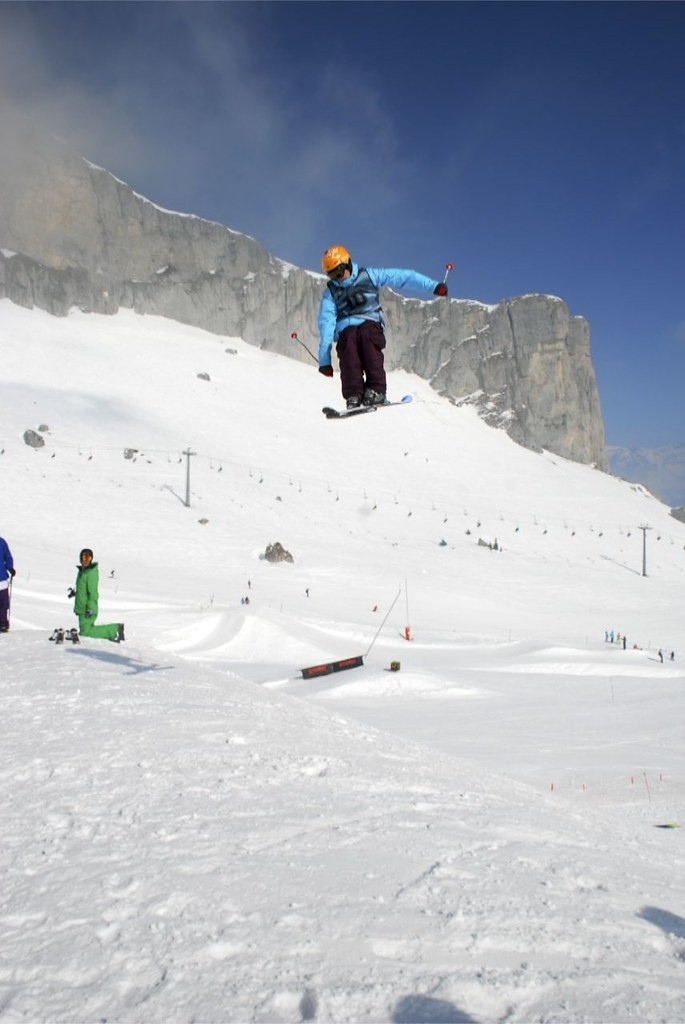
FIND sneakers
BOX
[120,623,125,641]
[363,389,385,406]
[114,624,121,643]
[346,395,361,407]
[0,625,8,631]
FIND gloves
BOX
[9,569,15,577]
[85,611,91,618]
[434,282,448,297]
[318,365,334,378]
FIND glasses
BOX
[322,265,350,280]
[81,555,92,561]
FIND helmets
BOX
[321,246,351,272]
[80,549,93,556]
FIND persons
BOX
[0,536,15,632]
[67,548,126,644]
[315,244,448,411]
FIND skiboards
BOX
[48,627,80,644]
[322,396,409,419]
